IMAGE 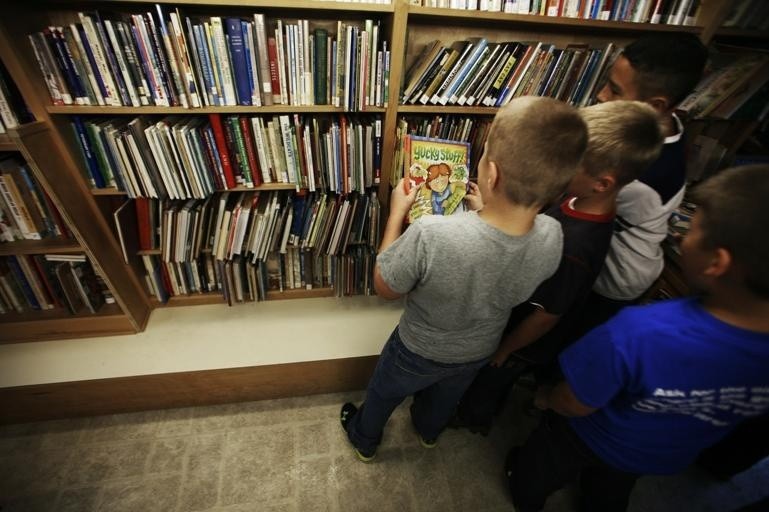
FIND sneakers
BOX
[410,402,439,449]
[504,442,546,512]
[339,401,377,462]
[574,469,598,510]
[446,399,498,431]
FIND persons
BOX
[505,163,768,509]
[460,102,665,435]
[340,95,589,464]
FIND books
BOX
[401,38,769,128]
[29,2,390,308]
[1,69,115,314]
[394,117,492,229]
[408,1,769,30]
